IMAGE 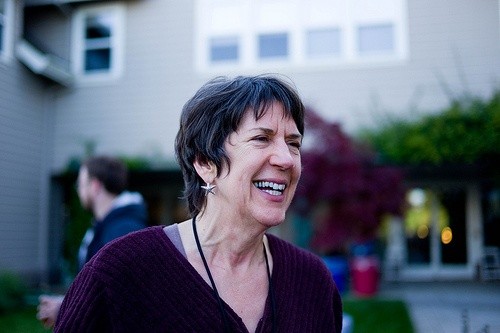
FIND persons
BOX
[52,75,343,333]
[37,153,150,329]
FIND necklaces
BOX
[192,215,278,333]
